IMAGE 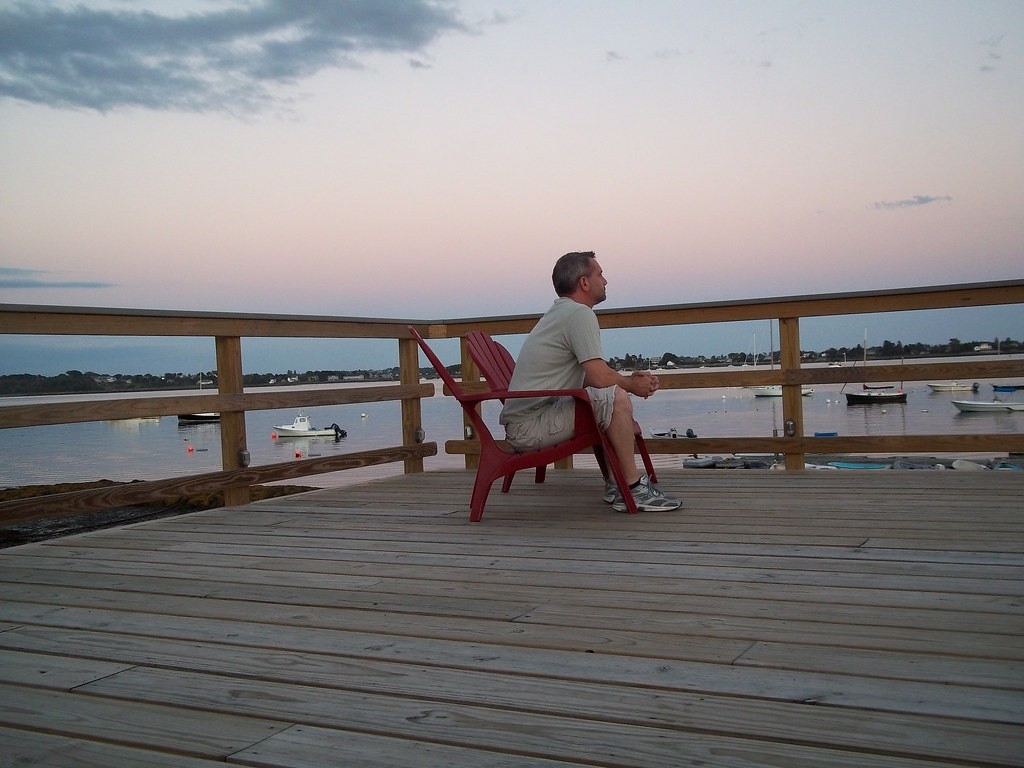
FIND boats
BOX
[951,399,1024,412]
[682,452,1023,469]
[992,384,1018,393]
[272,413,341,437]
[927,379,979,391]
[648,424,697,439]
[177,413,220,427]
[863,384,895,390]
[749,319,813,397]
[845,391,908,406]
[828,361,842,366]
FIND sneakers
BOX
[602,478,619,504]
[612,473,683,512]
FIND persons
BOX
[499,251,682,513]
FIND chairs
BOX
[408,323,658,522]
